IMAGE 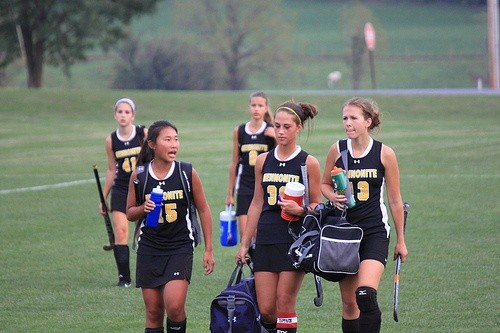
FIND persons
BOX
[224,92,275,245]
[98,98,151,288]
[322,97,410,333]
[234,101,323,333]
[125,120,214,333]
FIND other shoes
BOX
[118,280,131,287]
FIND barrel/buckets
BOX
[280,182,304,222]
[219,203,238,247]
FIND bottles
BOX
[330,165,355,209]
[145,185,164,227]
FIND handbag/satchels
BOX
[209,257,263,333]
[288,199,364,283]
[219,203,238,246]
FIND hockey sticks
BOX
[93,168,116,251]
[392,202,411,323]
[301,165,324,306]
